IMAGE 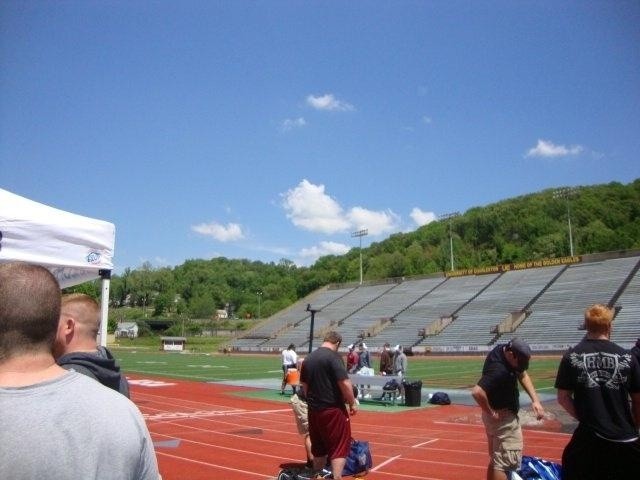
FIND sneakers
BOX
[358,393,406,400]
[304,462,313,468]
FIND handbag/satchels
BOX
[329,439,371,476]
[510,456,561,480]
[383,380,398,391]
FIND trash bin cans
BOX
[404,381,422,406]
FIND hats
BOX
[384,343,402,350]
[505,338,531,370]
[347,342,368,349]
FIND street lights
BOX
[552,185,580,257]
[351,229,368,285]
[440,210,460,272]
[256,288,263,320]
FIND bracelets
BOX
[492,411,496,417]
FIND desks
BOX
[348,374,405,403]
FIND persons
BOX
[554,303,640,480]
[471,338,545,480]
[0,261,162,480]
[281,331,372,476]
[379,343,407,377]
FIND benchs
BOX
[358,387,398,408]
